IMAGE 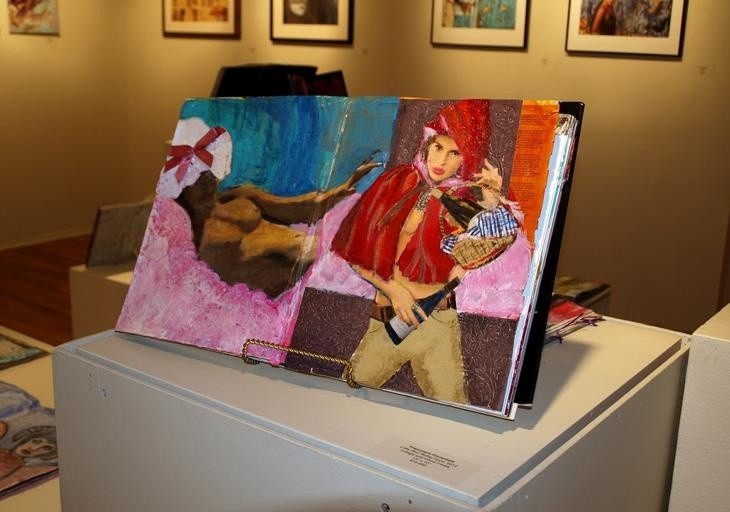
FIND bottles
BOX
[430,185,487,232]
[383,275,462,348]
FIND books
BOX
[545,274,612,344]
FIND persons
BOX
[330,99,521,405]
[178,148,384,301]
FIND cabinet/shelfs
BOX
[49,259,729,512]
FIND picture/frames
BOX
[430,1,529,52]
[564,1,690,62]
[269,1,353,47]
[161,0,240,42]
